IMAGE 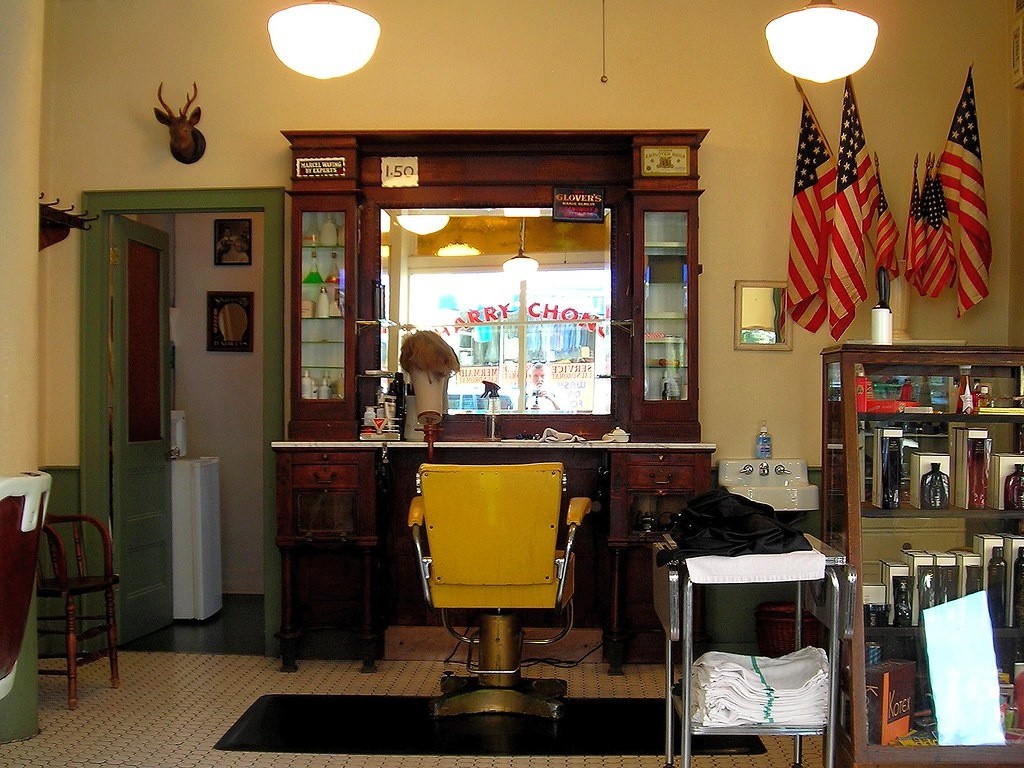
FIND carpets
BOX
[212,693,767,755]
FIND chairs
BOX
[409,461,593,718]
[35,512,120,710]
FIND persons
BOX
[526,364,562,410]
[219,222,248,251]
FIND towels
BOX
[691,644,830,729]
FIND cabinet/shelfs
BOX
[626,137,707,441]
[286,138,365,442]
[820,344,1024,768]
[653,531,859,768]
[272,445,713,676]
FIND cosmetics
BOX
[857,366,1023,628]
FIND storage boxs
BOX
[899,549,926,564]
[986,452,1024,510]
[948,422,988,508]
[932,554,958,567]
[1003,536,1024,627]
[872,426,904,506]
[957,553,981,599]
[885,564,911,625]
[908,554,936,626]
[862,583,887,607]
[978,535,1004,591]
[973,534,991,554]
[910,453,951,509]
[910,452,932,506]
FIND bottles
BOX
[363,371,406,434]
[855,364,950,413]
[968,438,987,509]
[1013,547,1024,627]
[303,250,344,318]
[988,547,1007,628]
[864,595,893,628]
[858,419,867,435]
[921,463,949,510]
[925,570,936,609]
[950,364,990,414]
[1004,464,1024,510]
[966,565,983,596]
[894,580,911,627]
[945,569,956,601]
[301,369,345,400]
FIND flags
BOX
[787,65,993,342]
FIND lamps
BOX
[266,0,381,81]
[502,216,540,283]
[763,0,879,84]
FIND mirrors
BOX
[734,279,795,351]
[205,291,254,352]
[379,208,611,414]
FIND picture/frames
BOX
[213,218,252,266]
